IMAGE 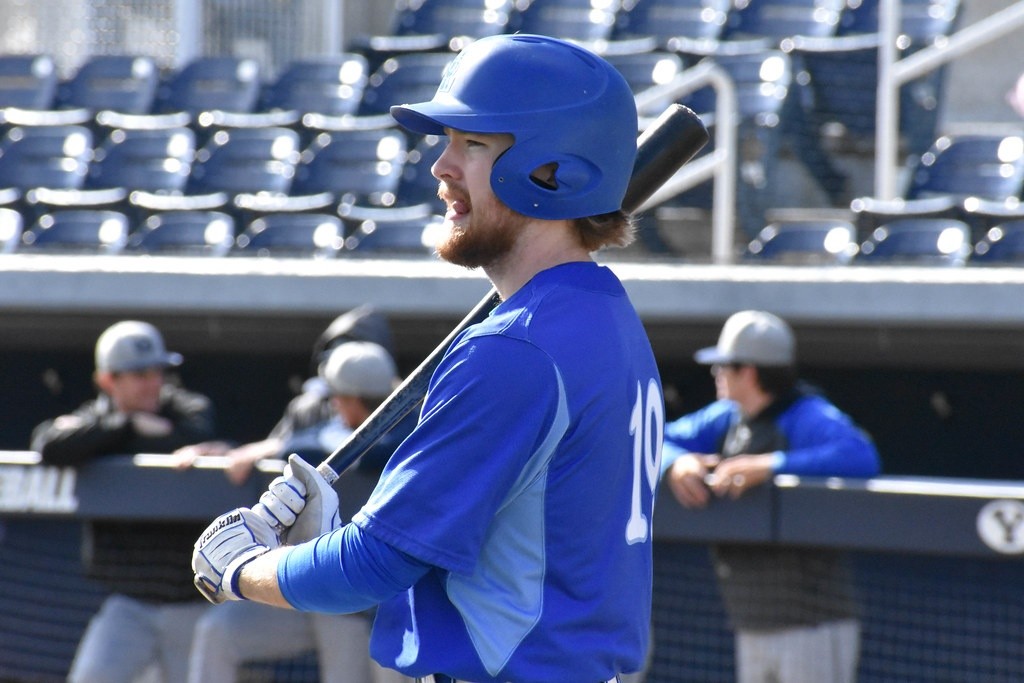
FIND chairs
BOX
[0,1,1024,266]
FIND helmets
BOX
[390,34,639,219]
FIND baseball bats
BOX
[193,103,711,604]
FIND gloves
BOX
[252,453,342,546]
[191,507,282,605]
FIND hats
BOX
[94,322,183,371]
[693,311,795,368]
[312,339,402,399]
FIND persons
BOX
[175,306,403,483]
[658,308,880,682]
[32,322,264,683]
[186,34,662,683]
[190,341,412,683]
